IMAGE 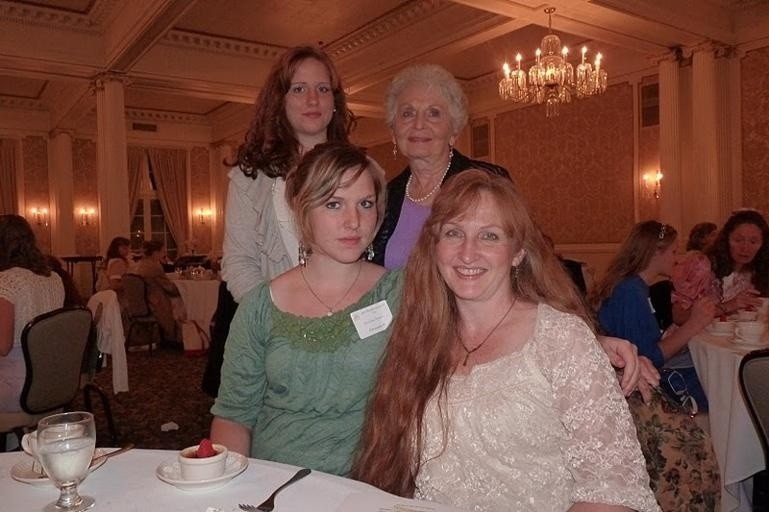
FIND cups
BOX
[175,267,182,279]
[179,445,228,480]
[37,411,96,511]
[734,321,765,341]
[21,424,83,473]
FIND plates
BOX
[11,447,106,486]
[728,336,768,346]
[155,451,249,490]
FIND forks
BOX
[239,469,310,511]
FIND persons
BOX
[0,213,67,452]
[670,209,769,326]
[221,44,350,305]
[209,140,406,480]
[132,237,180,328]
[104,236,132,290]
[587,219,717,413]
[347,168,663,512]
[686,221,718,251]
[365,64,662,406]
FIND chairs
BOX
[119,272,168,359]
[737,347,769,468]
[1,302,94,452]
[76,289,119,447]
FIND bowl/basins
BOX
[706,322,735,336]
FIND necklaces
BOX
[299,262,363,317]
[455,296,517,367]
[404,160,451,203]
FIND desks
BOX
[59,254,104,297]
